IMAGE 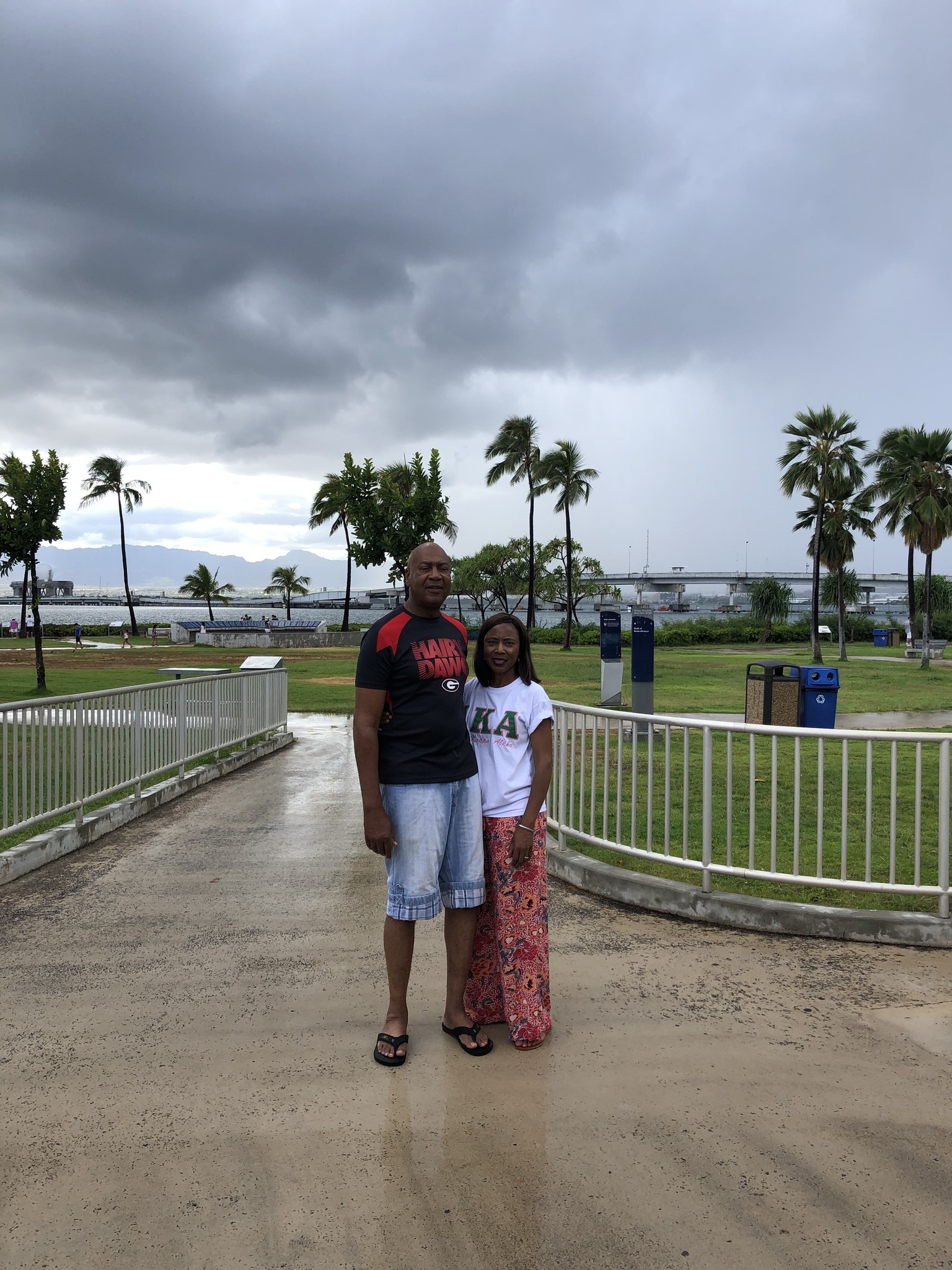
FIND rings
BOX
[525,857,529,859]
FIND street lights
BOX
[872,540,875,575]
[628,546,631,574]
[745,541,748,572]
[107,583,108,597]
[84,583,86,595]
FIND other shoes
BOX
[121,647,124,648]
[152,645,155,646]
[906,643,909,645]
[130,645,134,648]
[909,643,911,644]
[155,644,159,646]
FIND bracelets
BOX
[518,824,536,832]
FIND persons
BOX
[26,615,34,638]
[271,615,278,620]
[462,612,557,1051]
[902,617,912,646]
[121,628,133,648]
[249,616,253,620]
[352,541,494,1065]
[262,616,265,620]
[265,617,272,633]
[74,623,84,648]
[239,617,243,621]
[245,615,250,620]
[152,624,159,646]
[10,618,18,638]
[200,622,206,633]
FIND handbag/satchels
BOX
[9,629,11,632]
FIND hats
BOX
[12,618,15,619]
[265,617,270,621]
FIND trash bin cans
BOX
[887,627,900,647]
[744,660,801,726]
[790,665,840,730]
[872,629,888,647]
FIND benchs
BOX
[904,639,948,660]
[155,667,232,693]
[120,626,147,637]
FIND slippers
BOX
[442,1019,493,1057]
[515,1025,552,1051]
[374,1033,409,1067]
